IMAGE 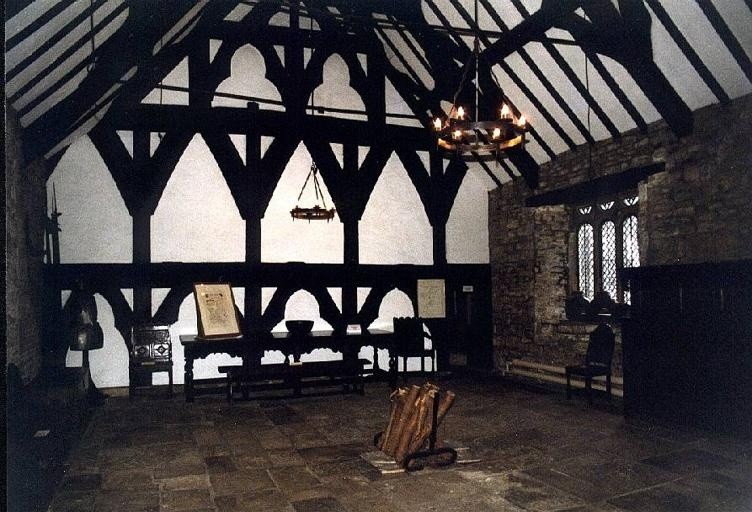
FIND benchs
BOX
[218,357,371,406]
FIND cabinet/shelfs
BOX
[615,261,752,438]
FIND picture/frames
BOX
[191,280,242,341]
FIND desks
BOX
[178,328,394,403]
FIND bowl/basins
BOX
[286,320,314,333]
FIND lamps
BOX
[289,2,337,222]
[430,1,528,166]
[69,303,109,405]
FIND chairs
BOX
[564,321,616,409]
[124,326,174,399]
[391,314,436,385]
